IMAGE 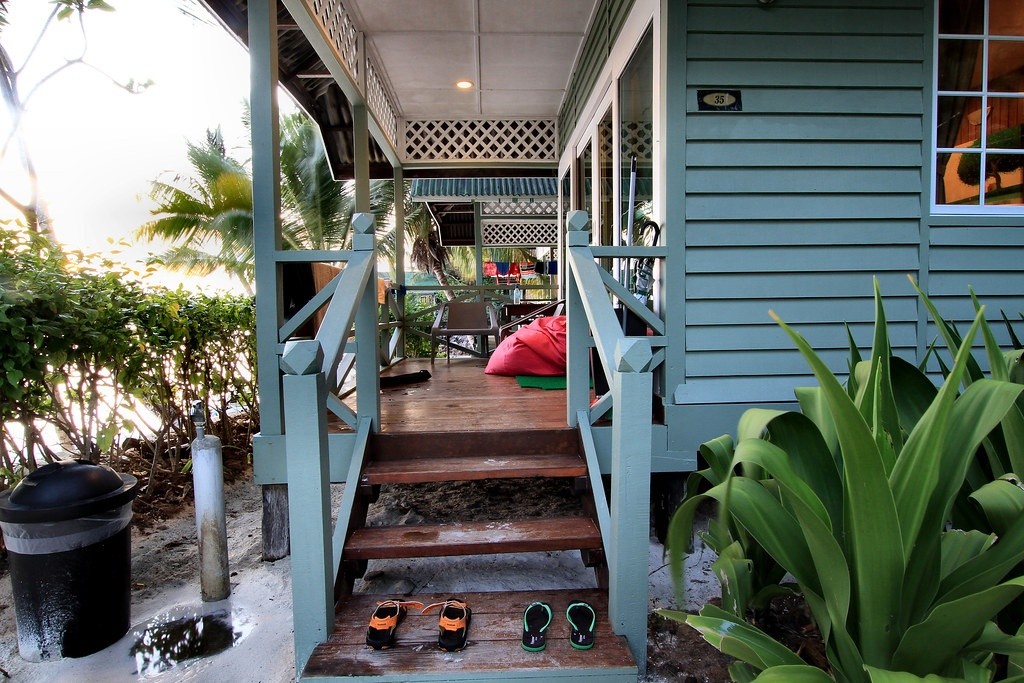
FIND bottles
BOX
[513,286,520,304]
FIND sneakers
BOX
[420,597,472,651]
[365,598,424,649]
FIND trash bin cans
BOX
[0,462,141,663]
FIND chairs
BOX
[431,303,500,365]
[499,298,566,344]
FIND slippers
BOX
[565,600,597,650]
[520,601,553,652]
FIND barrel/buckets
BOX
[0,458,139,663]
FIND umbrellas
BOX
[635,222,659,306]
